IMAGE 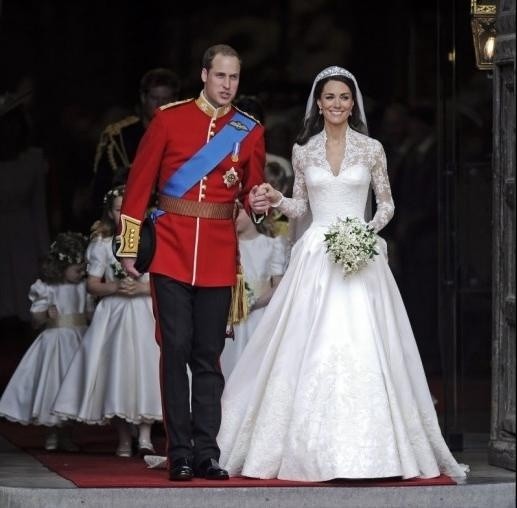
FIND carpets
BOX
[2,408,455,488]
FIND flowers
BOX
[323,214,380,275]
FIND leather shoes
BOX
[168,464,193,481]
[41,431,155,458]
[194,457,230,481]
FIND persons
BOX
[0,44,438,485]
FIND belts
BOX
[154,191,240,222]
